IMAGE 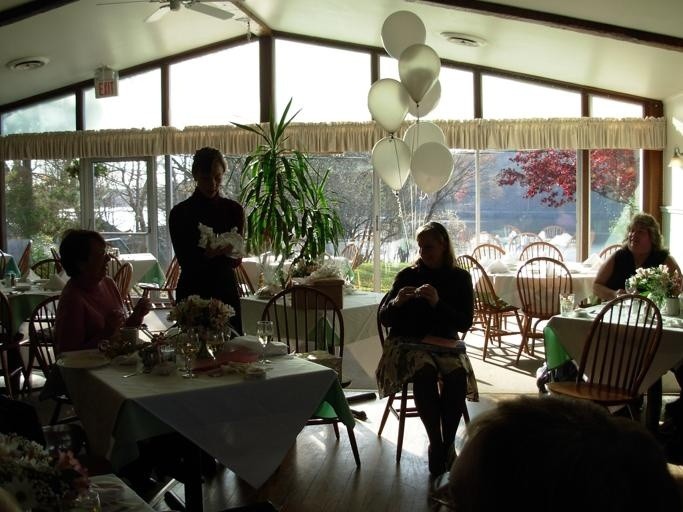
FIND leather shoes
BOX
[427,442,457,476]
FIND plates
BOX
[56,352,113,369]
[4,279,55,294]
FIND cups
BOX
[560,291,575,318]
[625,277,637,296]
[121,327,140,343]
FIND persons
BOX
[590,214,682,304]
[377,222,475,476]
[166,147,247,475]
[51,225,158,490]
[426,392,683,511]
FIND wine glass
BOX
[180,330,226,378]
[255,321,274,366]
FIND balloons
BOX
[399,67,441,121]
[379,9,426,64]
[370,134,412,193]
[409,141,455,197]
[396,43,441,104]
[401,120,445,157]
[366,77,409,134]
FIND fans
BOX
[95,0,248,23]
